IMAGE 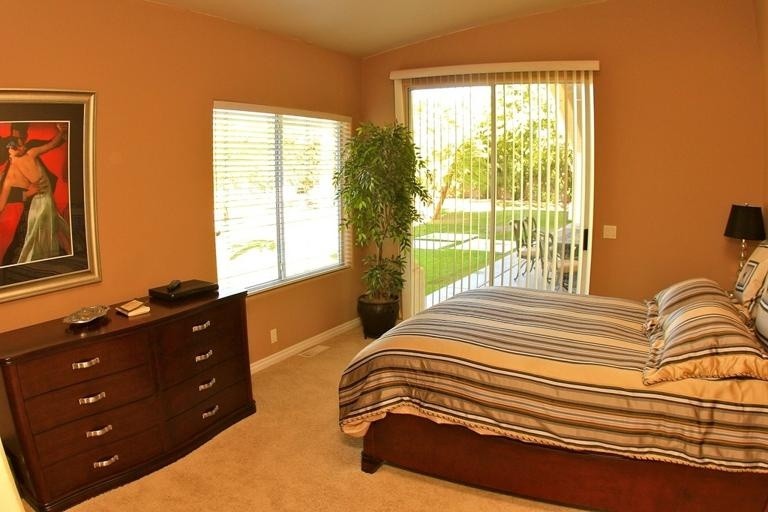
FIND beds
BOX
[340,231,767,512]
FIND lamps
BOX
[723,203,766,278]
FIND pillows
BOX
[732,239,768,313]
[749,277,768,349]
[644,276,754,337]
[643,300,765,387]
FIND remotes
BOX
[166,280,180,292]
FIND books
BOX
[115,298,151,317]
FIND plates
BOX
[64,304,110,324]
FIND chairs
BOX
[539,231,571,277]
[507,218,554,280]
[551,250,578,292]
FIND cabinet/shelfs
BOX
[0,278,257,510]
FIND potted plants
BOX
[335,119,433,339]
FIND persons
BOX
[1,124,71,265]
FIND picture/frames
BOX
[0,87,104,305]
